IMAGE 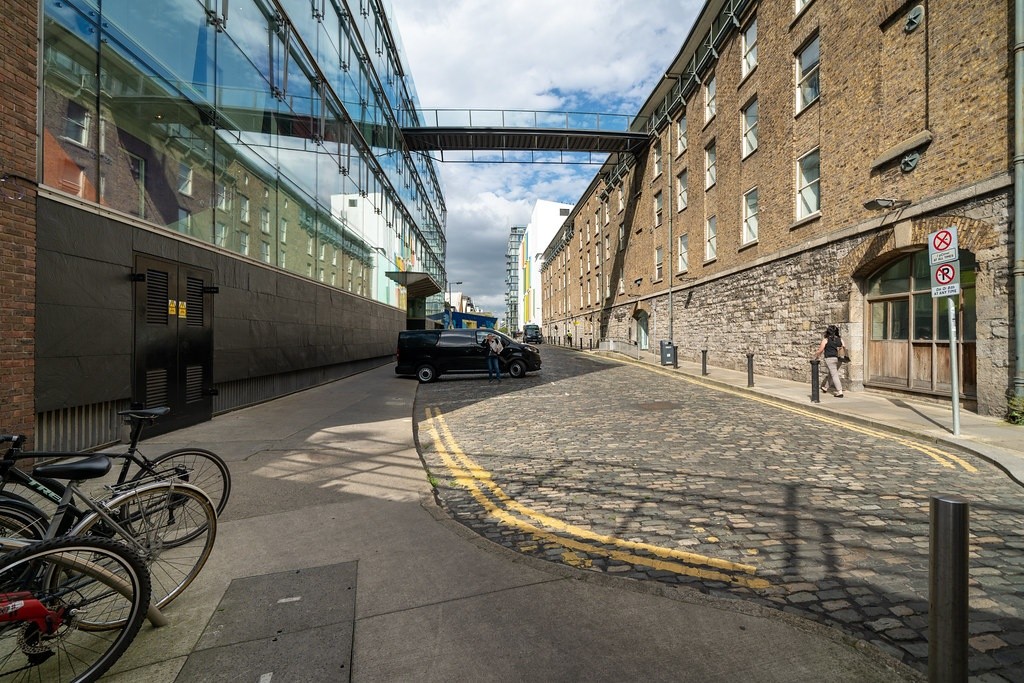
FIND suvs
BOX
[523,325,543,344]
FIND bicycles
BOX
[0,406,230,682]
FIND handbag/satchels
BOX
[836,346,850,364]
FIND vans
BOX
[395,328,542,384]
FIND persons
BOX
[816,325,845,398]
[481,334,501,382]
[567,330,572,345]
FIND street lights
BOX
[449,281,463,329]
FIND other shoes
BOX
[820,387,827,393]
[834,394,843,398]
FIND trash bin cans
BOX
[660,338,674,366]
